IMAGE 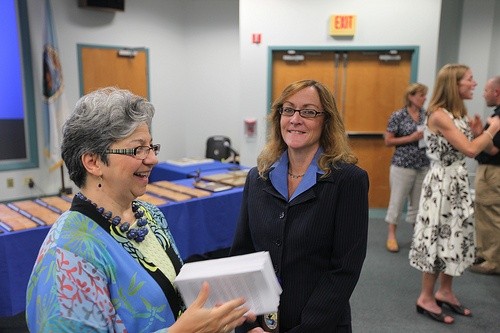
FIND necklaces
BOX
[288,172,304,179]
[75,191,148,242]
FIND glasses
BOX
[277,105,325,119]
[106,143,160,160]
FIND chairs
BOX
[207,136,240,165]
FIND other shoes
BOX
[471,257,500,275]
[387,239,399,252]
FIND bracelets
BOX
[482,130,493,139]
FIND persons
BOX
[385,83,430,252]
[407,65,500,323]
[466,74,500,274]
[230,79,370,333]
[25,85,265,333]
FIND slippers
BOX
[436,299,473,317]
[416,303,456,323]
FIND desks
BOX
[0,160,254,315]
